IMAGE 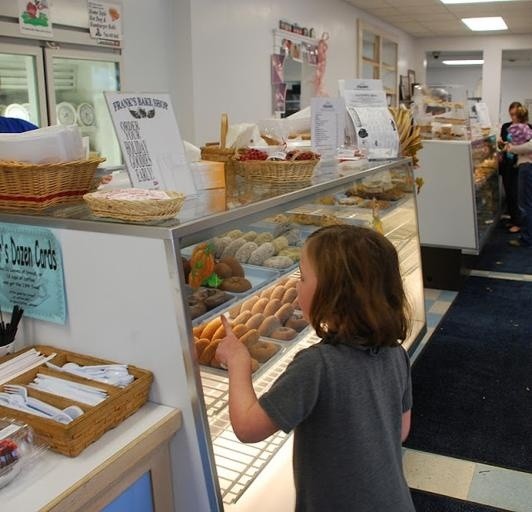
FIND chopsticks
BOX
[1,301,24,347]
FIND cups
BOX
[482,127,490,136]
[0,340,15,361]
[441,123,452,135]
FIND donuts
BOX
[181,229,308,372]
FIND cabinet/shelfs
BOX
[414,83,470,139]
[1,157,428,512]
[408,133,504,257]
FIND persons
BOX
[498,107,531,161]
[215,226,417,511]
[497,138,532,247]
[497,102,532,233]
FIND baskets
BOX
[200,113,320,184]
[82,190,185,221]
[1,343,154,457]
[1,156,106,213]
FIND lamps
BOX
[439,57,485,66]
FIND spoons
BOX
[43,359,130,387]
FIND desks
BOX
[0,402,183,512]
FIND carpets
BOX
[462,215,531,275]
[406,485,519,512]
[401,275,531,473]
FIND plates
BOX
[55,101,96,127]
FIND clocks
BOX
[78,102,97,126]
[55,102,77,127]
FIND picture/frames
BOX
[398,69,416,100]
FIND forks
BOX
[2,381,70,420]
